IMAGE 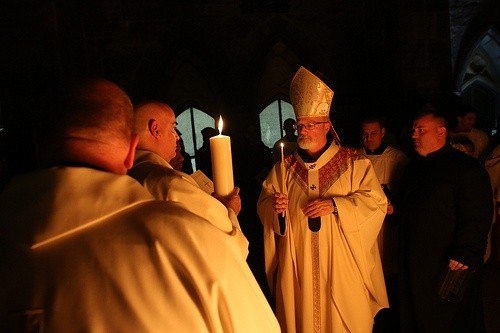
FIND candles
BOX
[209,114,235,196]
[280,142,284,218]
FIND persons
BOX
[355,116,405,266]
[170,102,500,299]
[0,77,282,333]
[128,101,242,233]
[387,114,495,333]
[256,66,389,333]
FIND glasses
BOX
[292,122,328,131]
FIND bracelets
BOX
[331,198,338,215]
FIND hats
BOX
[290,67,342,145]
